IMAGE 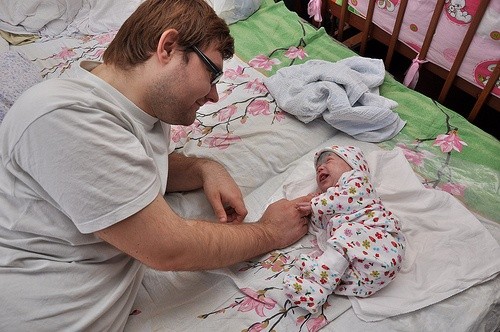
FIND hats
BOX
[310,143,368,169]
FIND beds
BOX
[0,1,500,331]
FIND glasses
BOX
[190,42,225,86]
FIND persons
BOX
[283,142,406,316]
[0,0,316,332]
